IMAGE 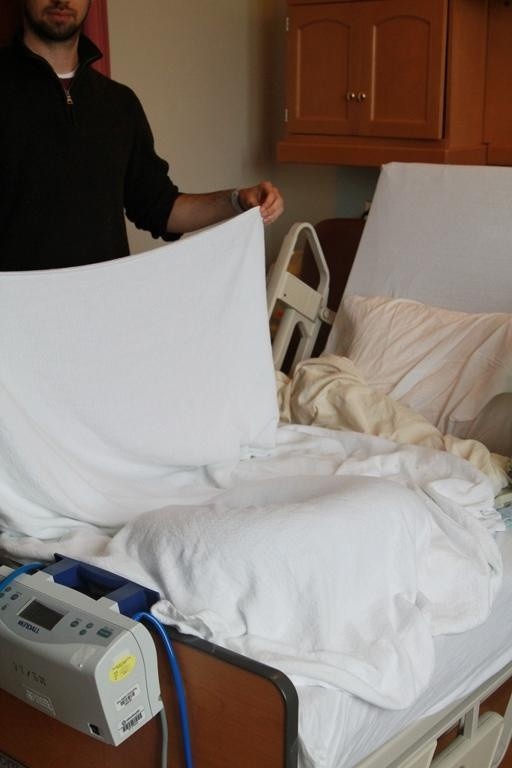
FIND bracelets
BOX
[230,186,249,215]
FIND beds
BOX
[0,161,511,768]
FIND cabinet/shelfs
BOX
[274,0,487,163]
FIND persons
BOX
[2,2,287,274]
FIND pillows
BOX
[344,295,512,436]
